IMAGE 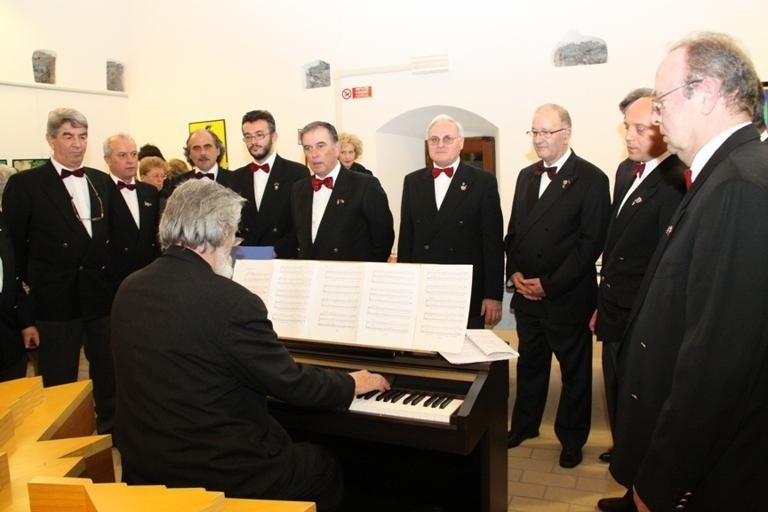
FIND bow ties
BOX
[631,163,646,177]
[312,175,335,191]
[682,168,693,187]
[194,172,214,180]
[117,181,137,191]
[533,163,558,178]
[250,162,269,173]
[431,165,453,177]
[60,168,86,178]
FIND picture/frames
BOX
[12,158,51,173]
[188,118,229,171]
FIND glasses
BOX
[242,132,271,143]
[73,193,106,222]
[429,136,459,146]
[221,233,245,248]
[524,129,565,137]
[651,78,700,112]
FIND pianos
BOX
[272,339,509,511]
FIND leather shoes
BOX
[507,426,540,448]
[599,447,614,461]
[597,495,635,511]
[559,441,584,469]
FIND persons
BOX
[505,103,613,468]
[224,109,312,248]
[591,25,768,512]
[107,176,393,511]
[395,112,504,329]
[139,157,170,191]
[164,158,189,181]
[0,163,41,386]
[335,132,377,176]
[160,126,233,225]
[273,121,395,262]
[1,104,132,440]
[102,132,160,271]
[136,143,165,162]
